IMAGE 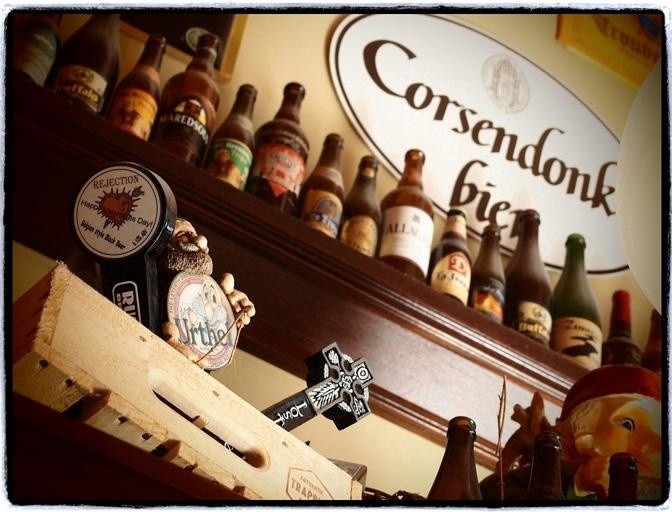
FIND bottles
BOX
[432,417,479,501]
[12,8,60,89]
[600,288,640,369]
[300,133,345,239]
[505,208,554,352]
[466,223,506,324]
[549,235,602,371]
[376,147,435,286]
[529,431,567,503]
[337,154,381,261]
[57,8,118,117]
[604,452,640,501]
[427,200,472,304]
[110,26,159,135]
[249,82,309,218]
[150,33,221,166]
[201,80,255,190]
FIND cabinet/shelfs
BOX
[6,68,611,509]
